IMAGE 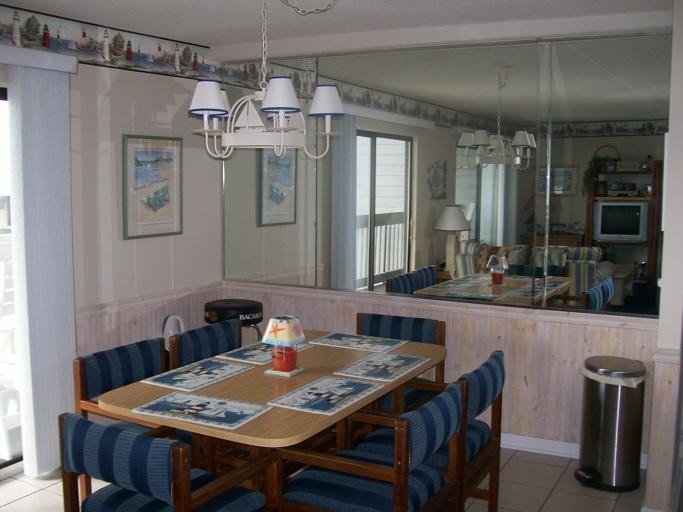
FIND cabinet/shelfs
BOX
[584,160,663,289]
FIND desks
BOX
[413,274,571,305]
[98,330,447,512]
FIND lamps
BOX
[188,0,345,160]
[457,63,537,171]
[433,206,471,272]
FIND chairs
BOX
[169,319,242,370]
[399,350,505,512]
[58,412,280,512]
[73,338,202,512]
[545,285,604,311]
[276,379,463,512]
[593,277,614,311]
[413,265,437,291]
[356,313,446,415]
[386,271,413,294]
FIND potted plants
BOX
[581,155,621,197]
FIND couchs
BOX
[455,240,602,297]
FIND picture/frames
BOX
[122,134,183,240]
[536,167,577,196]
[256,148,297,227]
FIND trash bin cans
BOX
[574,356,646,492]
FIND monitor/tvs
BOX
[592,201,648,243]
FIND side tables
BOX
[610,265,636,305]
